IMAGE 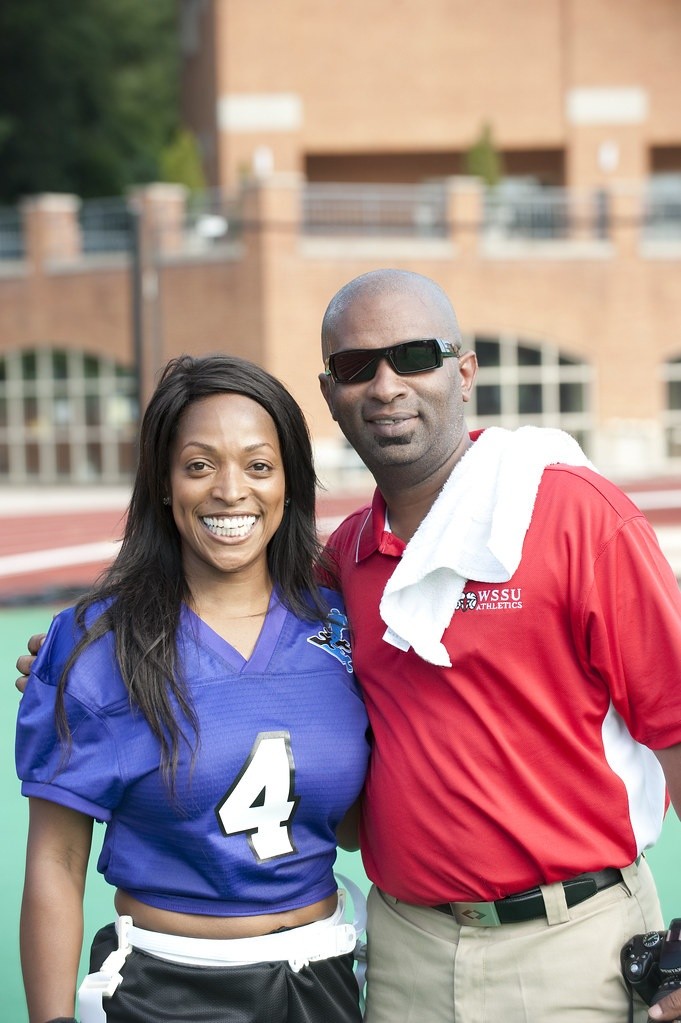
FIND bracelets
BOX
[47,1016,77,1023]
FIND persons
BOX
[15,349,371,1023]
[16,269,681,1023]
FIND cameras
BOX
[620,918,681,1023]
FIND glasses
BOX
[324,338,460,384]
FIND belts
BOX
[430,851,643,924]
